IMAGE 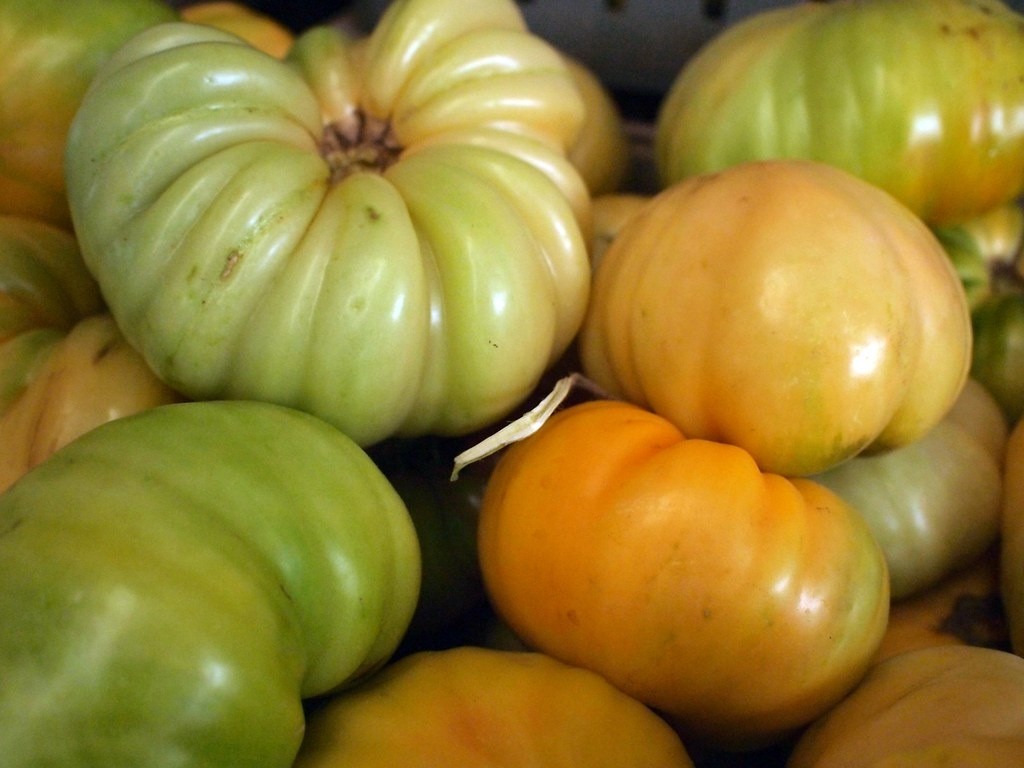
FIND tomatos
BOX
[0,0,1024,768]
